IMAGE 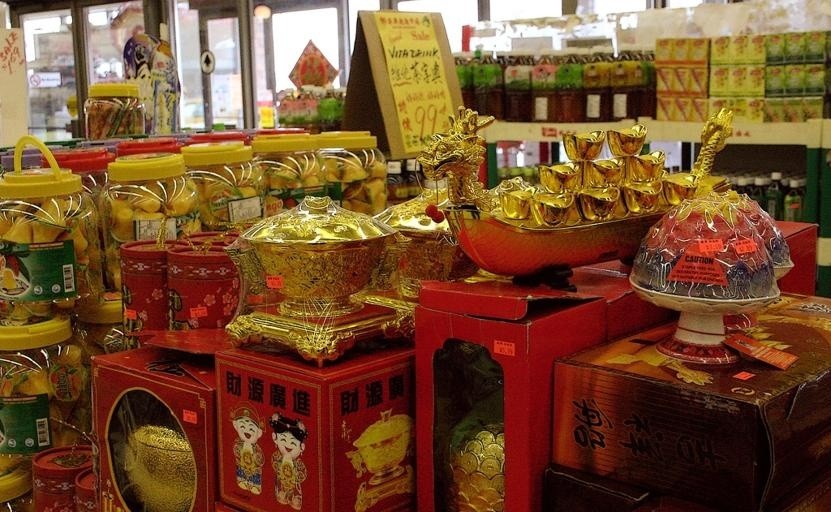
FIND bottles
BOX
[384,157,425,208]
[712,164,807,222]
[275,83,348,132]
[147,23,183,139]
[83,81,146,145]
[451,44,657,124]
[122,24,156,128]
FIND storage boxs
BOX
[91,329,414,511]
[414,226,829,510]
[120,233,245,341]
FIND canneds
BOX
[0,83,387,512]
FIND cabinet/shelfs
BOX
[471,114,831,297]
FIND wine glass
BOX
[718,257,797,333]
[626,265,783,365]
